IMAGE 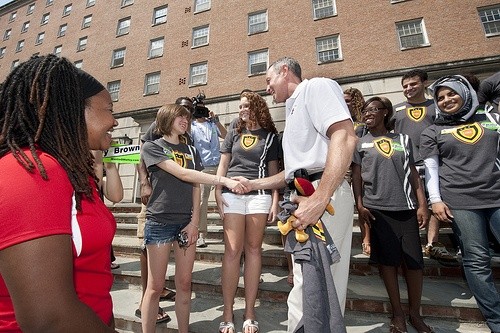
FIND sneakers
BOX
[424,241,457,260]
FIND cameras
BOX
[178,232,189,247]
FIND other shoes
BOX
[287,275,294,287]
[111,264,120,270]
[196,238,206,247]
[362,242,371,256]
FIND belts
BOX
[288,170,324,190]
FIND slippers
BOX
[160,288,177,302]
[219,313,235,333]
[390,316,407,333]
[135,306,169,323]
[408,318,436,333]
[242,314,260,333]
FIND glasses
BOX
[182,104,196,114]
[360,107,385,116]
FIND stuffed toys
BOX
[277,177,335,243]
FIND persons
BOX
[350,96,436,333]
[228,57,357,333]
[0,53,124,333]
[214,92,280,333]
[135,68,500,323]
[418,73,500,333]
[140,104,248,333]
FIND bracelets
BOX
[140,182,151,187]
[190,222,200,228]
[218,175,221,185]
